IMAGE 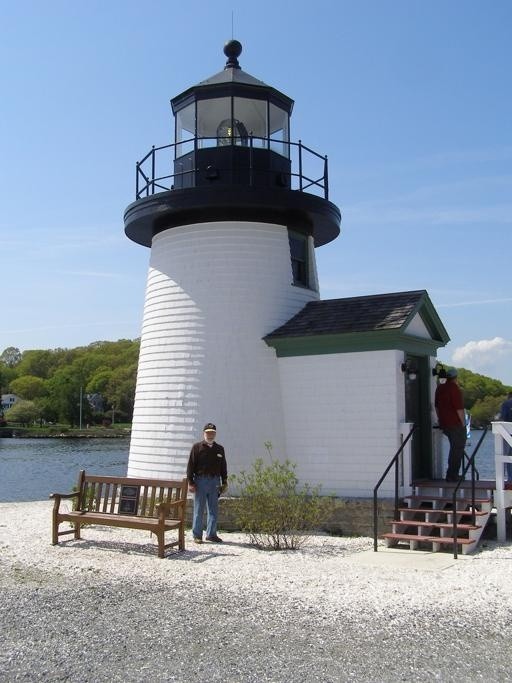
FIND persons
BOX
[496,391,512,483]
[434,368,466,481]
[187,422,228,544]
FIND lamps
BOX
[432,364,448,385]
[401,358,418,381]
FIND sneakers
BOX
[194,536,222,543]
[446,471,465,482]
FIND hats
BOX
[204,423,216,432]
[446,370,457,377]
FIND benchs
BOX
[49,471,188,557]
